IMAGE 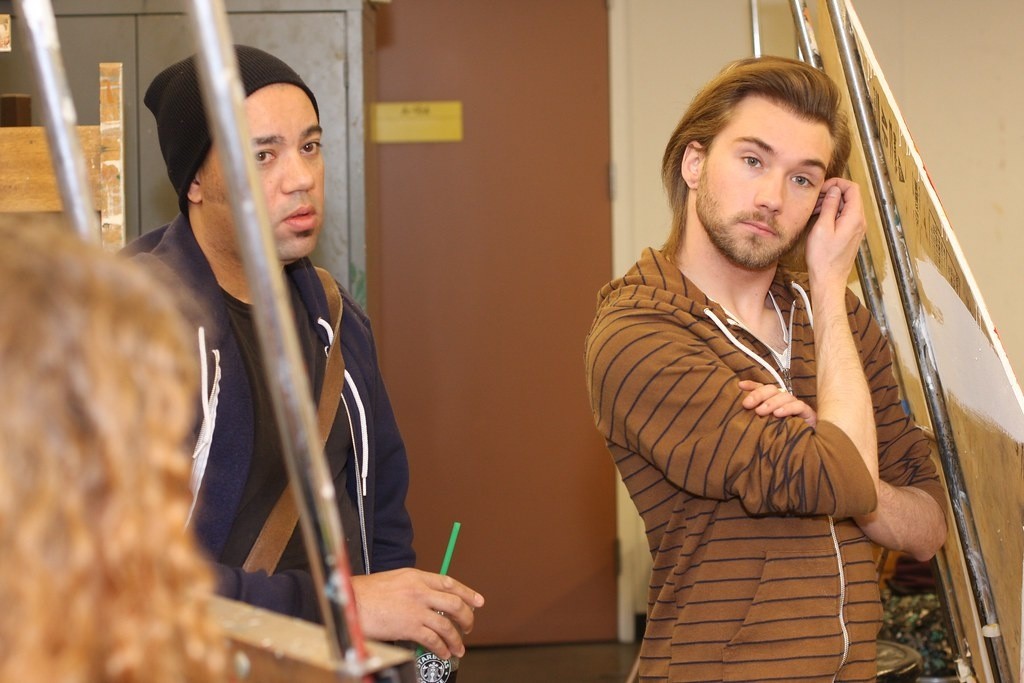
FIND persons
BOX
[87,45,486,683]
[583,54,948,683]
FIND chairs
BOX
[0,62,127,254]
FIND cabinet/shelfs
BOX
[0,0,394,317]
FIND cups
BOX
[394,606,475,683]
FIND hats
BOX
[142,44,322,218]
